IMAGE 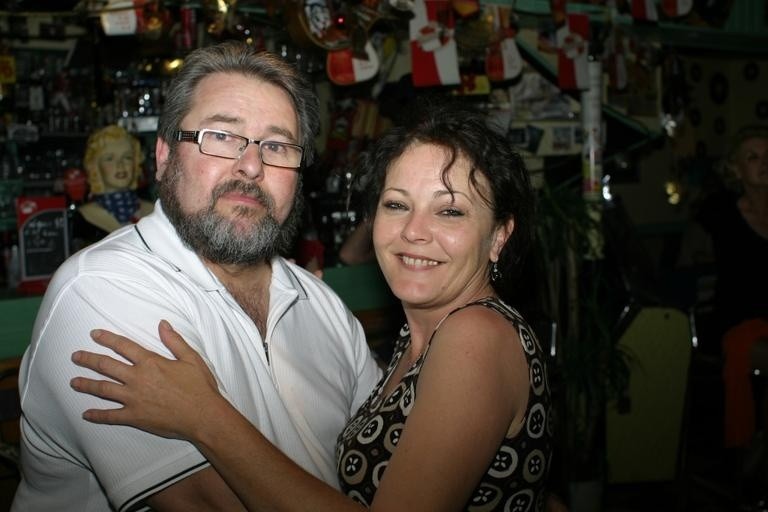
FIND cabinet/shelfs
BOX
[0,48,169,265]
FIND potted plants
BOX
[525,181,637,512]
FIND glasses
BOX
[172,129,306,171]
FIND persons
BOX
[73,124,157,246]
[701,124,767,490]
[10,44,387,510]
[69,103,556,509]
